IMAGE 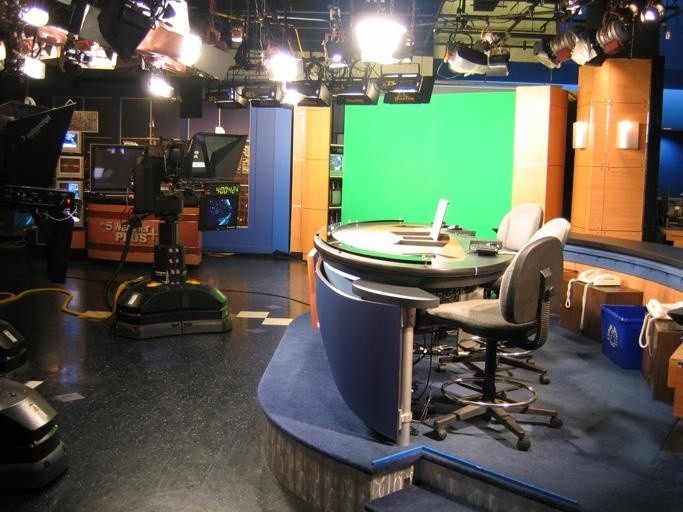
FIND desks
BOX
[561,269,683,418]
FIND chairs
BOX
[425,204,573,451]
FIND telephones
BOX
[646,298,683,321]
[577,269,621,286]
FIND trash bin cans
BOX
[600,304,648,369]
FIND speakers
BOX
[180,81,203,118]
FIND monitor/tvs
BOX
[89,144,147,193]
[201,196,238,231]
[183,140,207,169]
[56,130,84,227]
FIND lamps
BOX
[1,2,667,111]
